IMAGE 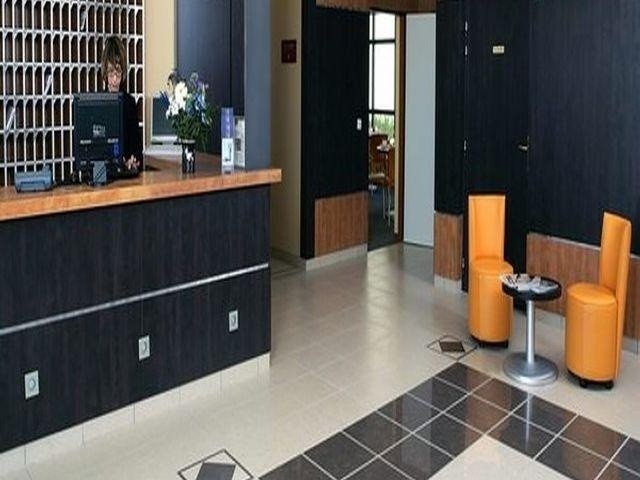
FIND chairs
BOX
[468,194,513,349]
[369,134,395,228]
[565,212,631,388]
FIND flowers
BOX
[161,69,221,152]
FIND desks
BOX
[502,276,561,386]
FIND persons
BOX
[100,36,143,172]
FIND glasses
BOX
[107,68,122,77]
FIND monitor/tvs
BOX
[74,93,123,183]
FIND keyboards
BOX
[74,163,139,180]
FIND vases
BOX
[181,139,196,173]
